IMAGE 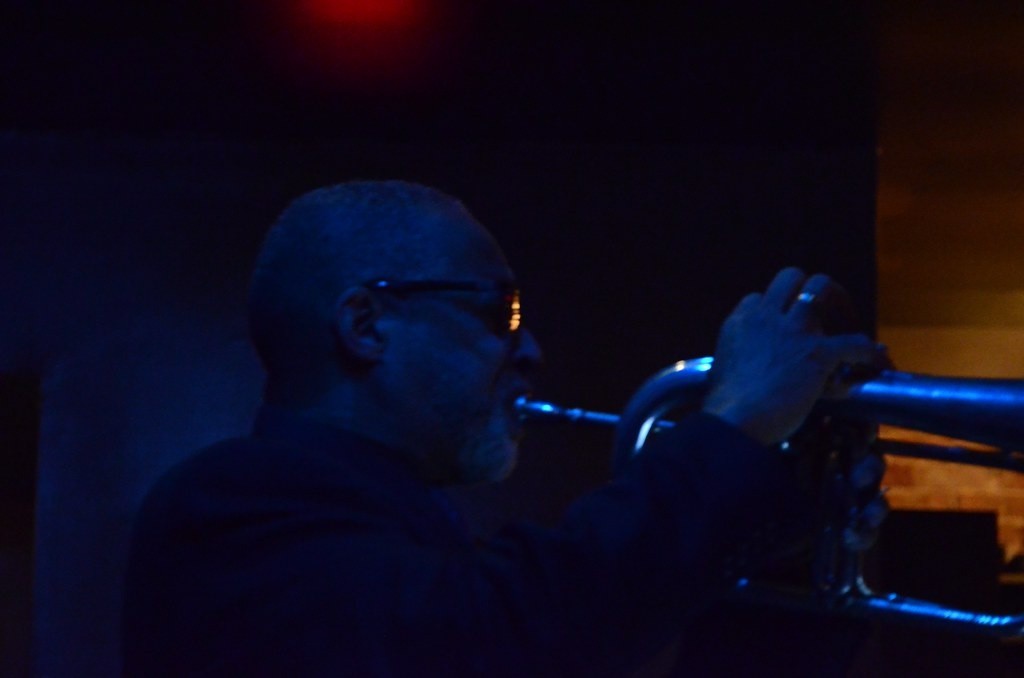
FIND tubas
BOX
[501,345,1024,648]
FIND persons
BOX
[116,179,891,677]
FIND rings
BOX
[791,287,819,308]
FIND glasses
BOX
[360,276,524,335]
